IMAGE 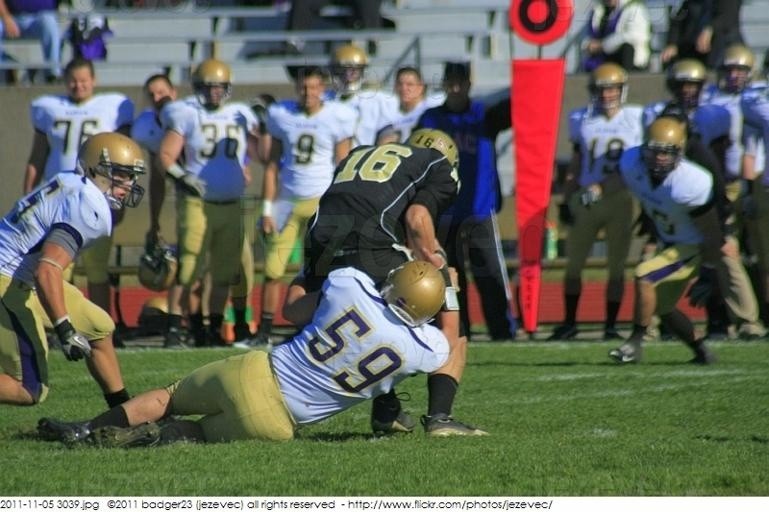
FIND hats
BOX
[441,57,474,85]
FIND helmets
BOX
[77,130,145,212]
[640,113,688,176]
[586,61,631,110]
[327,45,368,95]
[665,59,709,104]
[406,128,463,172]
[189,58,232,111]
[715,45,755,93]
[137,248,179,292]
[379,261,450,327]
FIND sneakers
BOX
[683,352,715,366]
[545,322,580,343]
[608,340,642,366]
[371,403,416,438]
[420,411,492,440]
[604,327,628,341]
[37,416,89,451]
[166,327,274,350]
[84,422,163,450]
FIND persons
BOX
[1,2,769,444]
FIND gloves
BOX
[176,172,209,199]
[685,269,720,309]
[579,184,601,211]
[54,321,94,363]
[250,96,269,134]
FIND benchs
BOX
[1,0,672,97]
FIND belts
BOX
[201,199,241,205]
